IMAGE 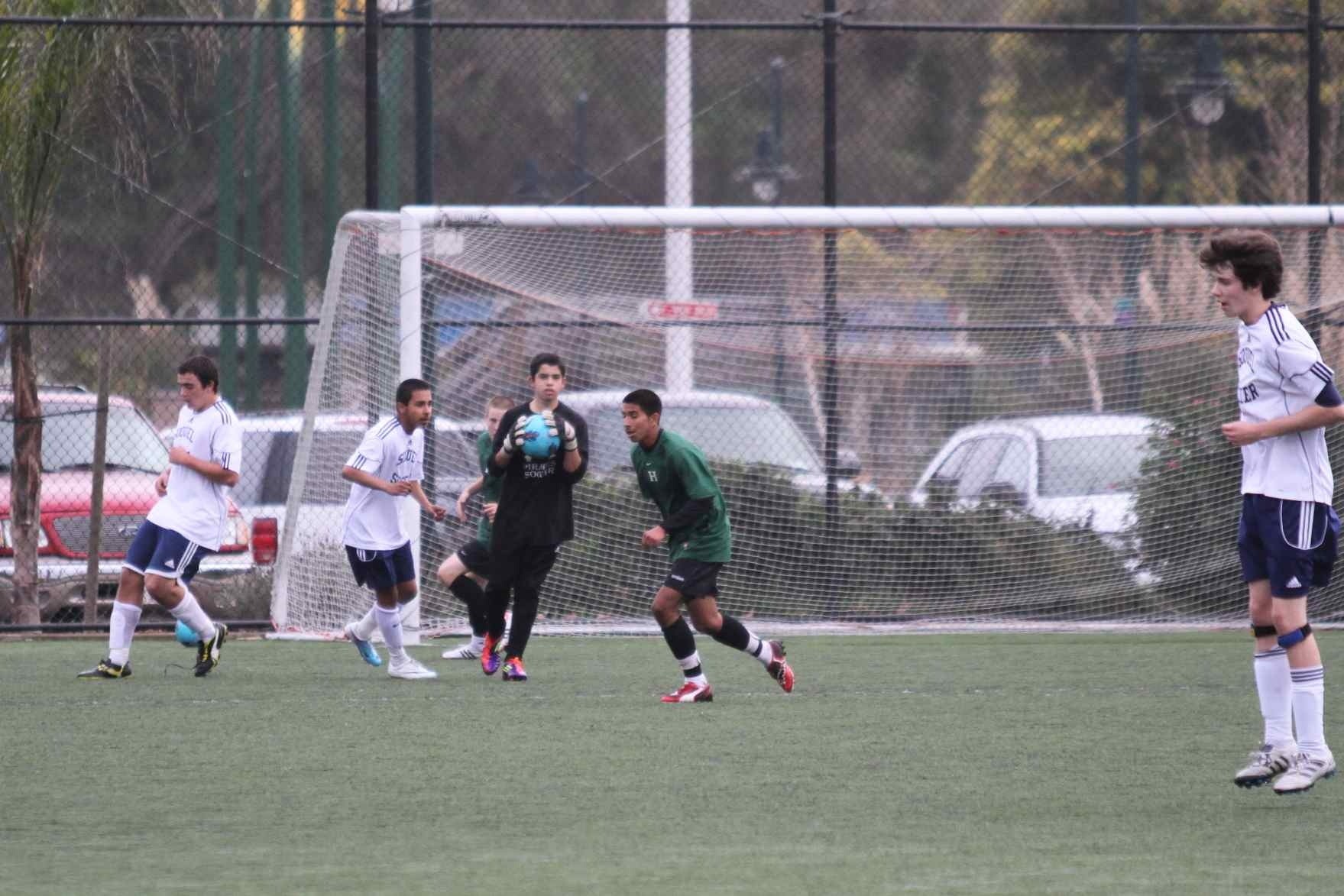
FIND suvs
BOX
[160,410,490,628]
[0,383,253,635]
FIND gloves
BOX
[500,414,526,458]
[541,409,577,451]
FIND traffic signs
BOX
[642,301,719,322]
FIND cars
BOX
[911,414,1178,591]
[558,389,890,518]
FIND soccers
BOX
[518,415,561,461]
[174,618,201,648]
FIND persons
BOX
[1196,229,1344,796]
[337,379,446,679]
[621,386,796,704]
[436,396,517,662]
[483,353,590,680]
[73,355,244,681]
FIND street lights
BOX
[750,61,789,411]
[1121,0,1228,418]
[365,0,408,425]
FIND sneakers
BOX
[482,630,505,675]
[1233,741,1297,789]
[195,622,228,677]
[1273,750,1336,795]
[441,643,483,659]
[76,657,135,679]
[387,658,439,680]
[502,656,528,682]
[661,681,714,704]
[767,639,795,694]
[343,621,382,667]
